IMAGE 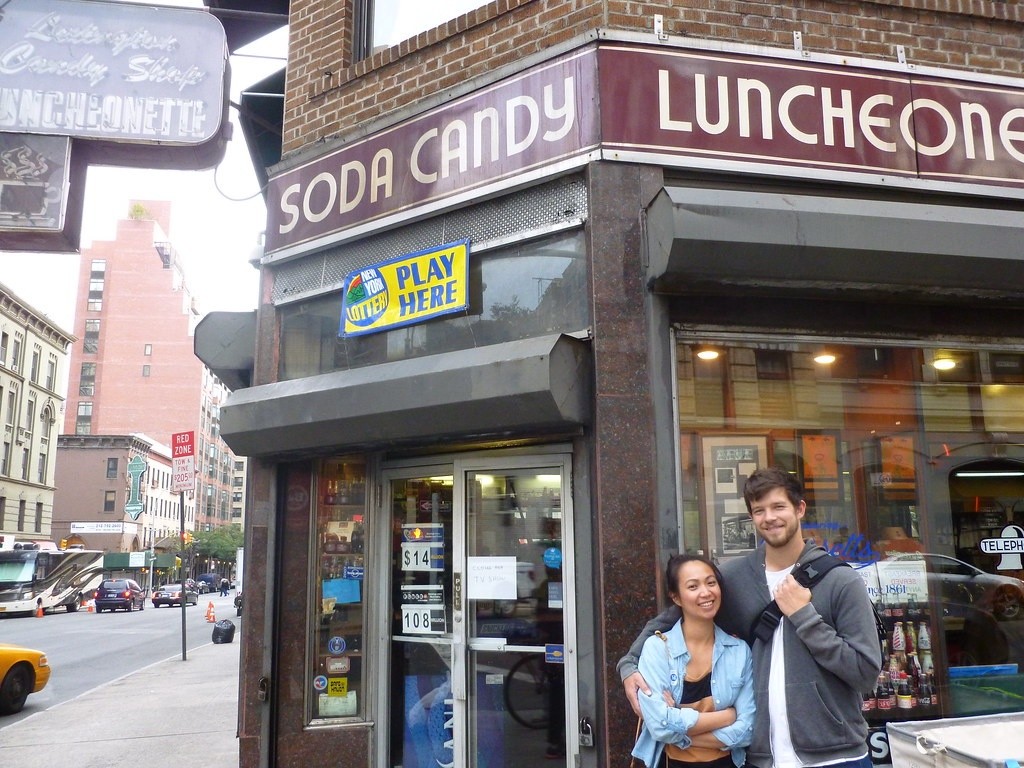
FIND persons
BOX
[631,556,757,768]
[220,576,230,597]
[616,468,882,768]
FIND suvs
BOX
[198,572,223,592]
[95,578,146,614]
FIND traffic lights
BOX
[61,539,67,548]
[186,533,193,544]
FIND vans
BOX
[475,562,537,620]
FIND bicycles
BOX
[500,650,571,732]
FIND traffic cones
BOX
[35,603,44,618]
[81,601,86,606]
[204,601,217,624]
[87,602,93,612]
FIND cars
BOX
[230,580,236,589]
[195,580,210,594]
[174,578,199,595]
[222,578,231,590]
[882,552,1024,622]
[152,583,198,608]
[0,642,50,716]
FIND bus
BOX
[0,550,107,618]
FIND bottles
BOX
[874,593,932,630]
[325,535,364,554]
[861,671,937,712]
[325,478,365,506]
[881,622,932,668]
[882,654,934,682]
